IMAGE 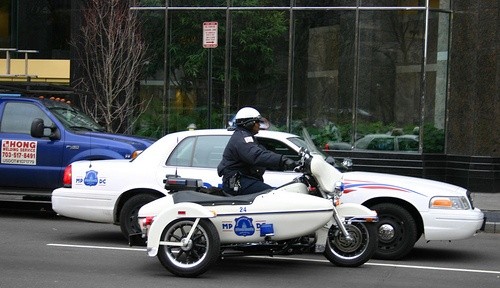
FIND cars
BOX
[50,122,485,261]
[323,128,420,153]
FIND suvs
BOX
[0,91,160,213]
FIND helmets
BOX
[234,107,264,122]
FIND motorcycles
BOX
[128,126,379,278]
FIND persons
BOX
[216,106,302,195]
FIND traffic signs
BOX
[202,21,219,49]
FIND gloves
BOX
[280,155,301,172]
